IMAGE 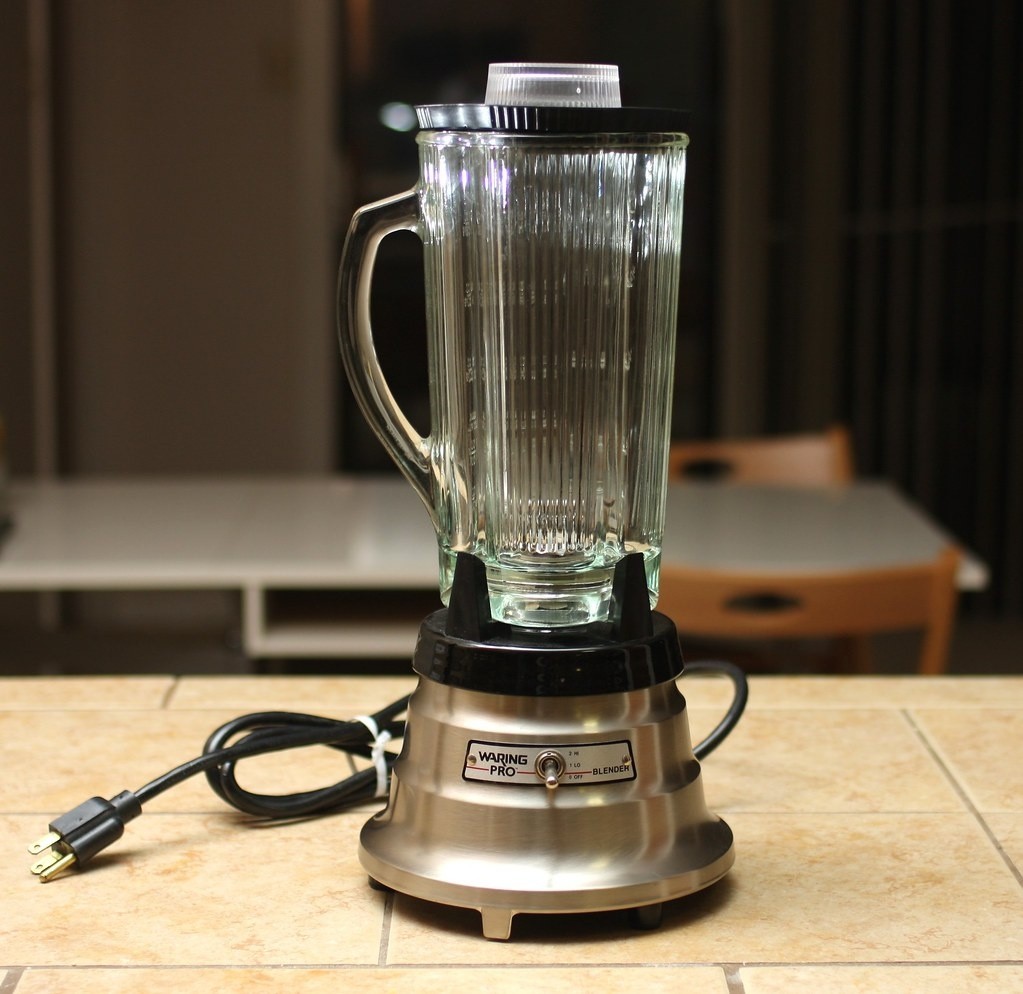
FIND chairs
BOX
[656,543,963,674]
[667,432,847,482]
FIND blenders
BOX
[331,56,738,943]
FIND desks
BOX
[1,482,983,674]
